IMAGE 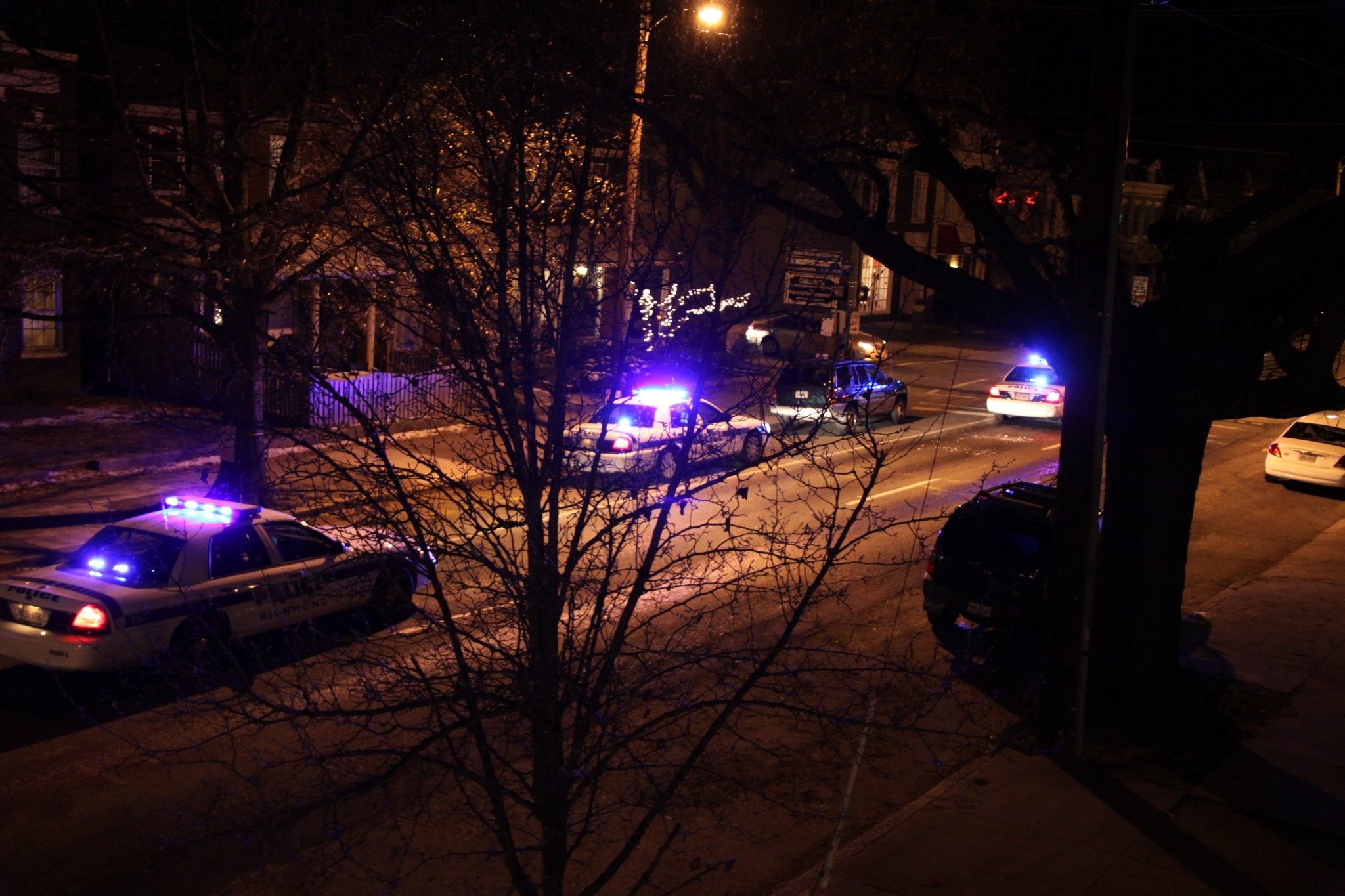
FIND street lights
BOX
[612,3,730,354]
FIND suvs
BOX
[768,358,910,435]
[923,483,1105,667]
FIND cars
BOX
[561,384,773,482]
[746,311,888,366]
[986,353,1066,422]
[2,494,436,684]
[1264,410,1345,492]
